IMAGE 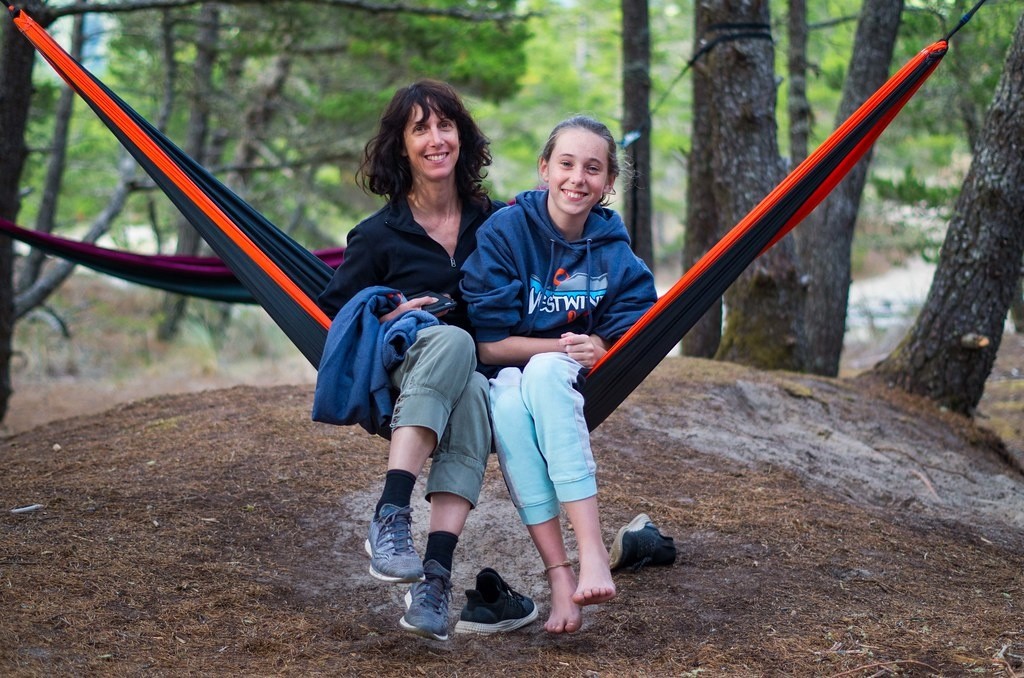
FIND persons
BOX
[458,117,658,633]
[317,78,507,642]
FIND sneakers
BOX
[398,560,453,642]
[608,514,674,571]
[455,568,539,637]
[364,503,425,583]
